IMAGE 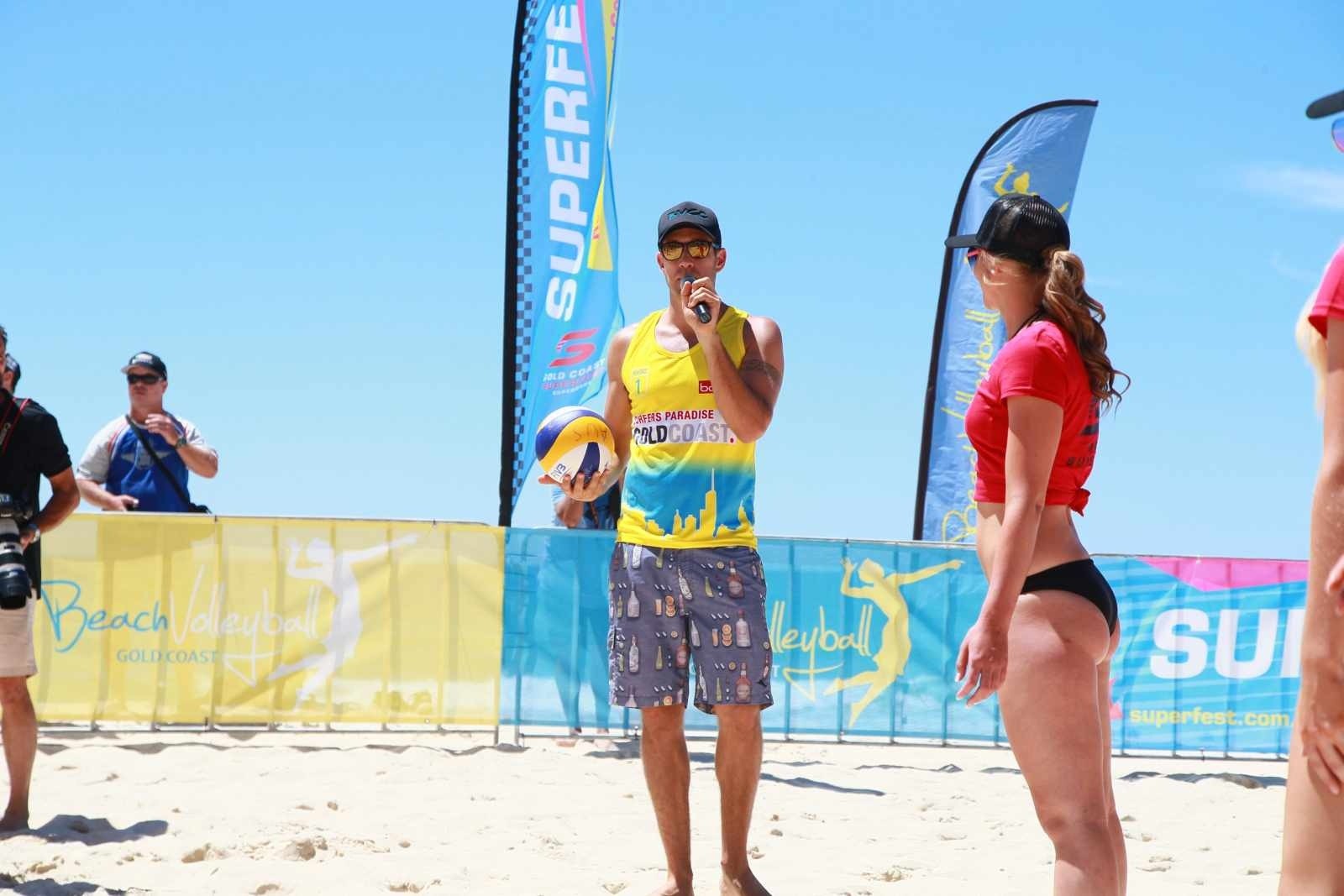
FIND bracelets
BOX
[28,523,40,544]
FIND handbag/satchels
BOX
[185,501,213,514]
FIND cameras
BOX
[0,508,33,609]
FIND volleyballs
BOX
[534,403,618,483]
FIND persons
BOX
[537,202,785,895]
[943,192,1130,896]
[1275,88,1344,896]
[0,325,219,831]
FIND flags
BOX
[916,100,1099,542]
[506,0,620,531]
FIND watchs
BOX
[175,436,187,449]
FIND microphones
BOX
[680,277,711,323]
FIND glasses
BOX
[127,374,165,384]
[661,241,712,260]
[966,246,985,269]
[1329,118,1344,152]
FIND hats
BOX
[122,351,171,380]
[4,353,24,382]
[1306,89,1344,119]
[944,193,1071,268]
[658,201,722,251]
